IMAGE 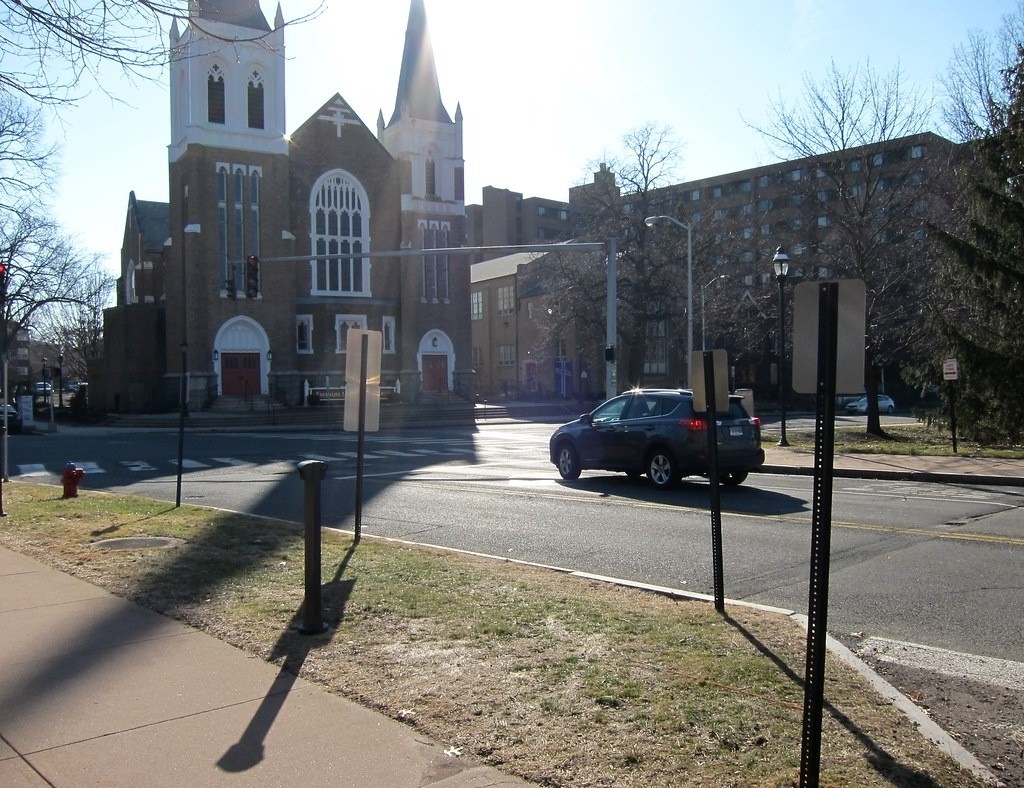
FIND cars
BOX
[0,403,23,436]
[35,382,54,396]
[65,381,80,392]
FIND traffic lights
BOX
[226,278,236,301]
[0,263,6,306]
[246,255,259,299]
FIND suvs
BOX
[846,394,895,415]
[548,389,766,494]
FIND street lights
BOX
[41,357,49,403]
[57,353,64,409]
[646,216,693,393]
[772,244,792,449]
[179,341,191,418]
[700,275,732,352]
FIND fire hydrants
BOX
[60,461,84,499]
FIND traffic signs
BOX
[943,358,957,381]
